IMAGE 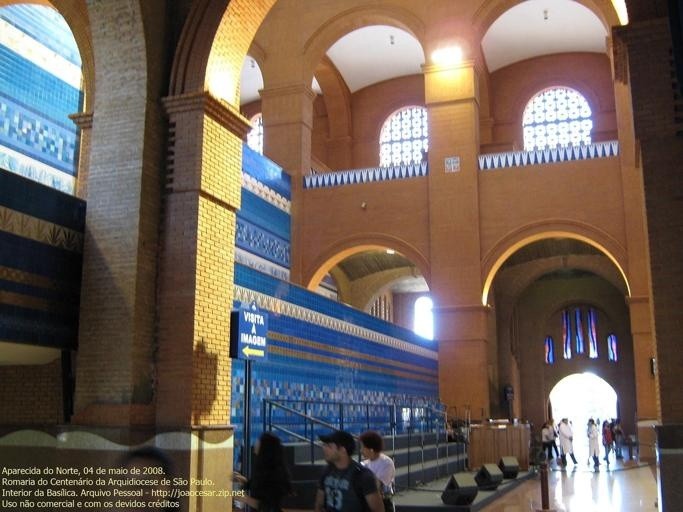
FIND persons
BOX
[522,416,627,467]
[359,431,396,510]
[233,434,285,511]
[121,448,182,511]
[443,418,468,443]
[314,430,384,510]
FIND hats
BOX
[318,432,356,456]
